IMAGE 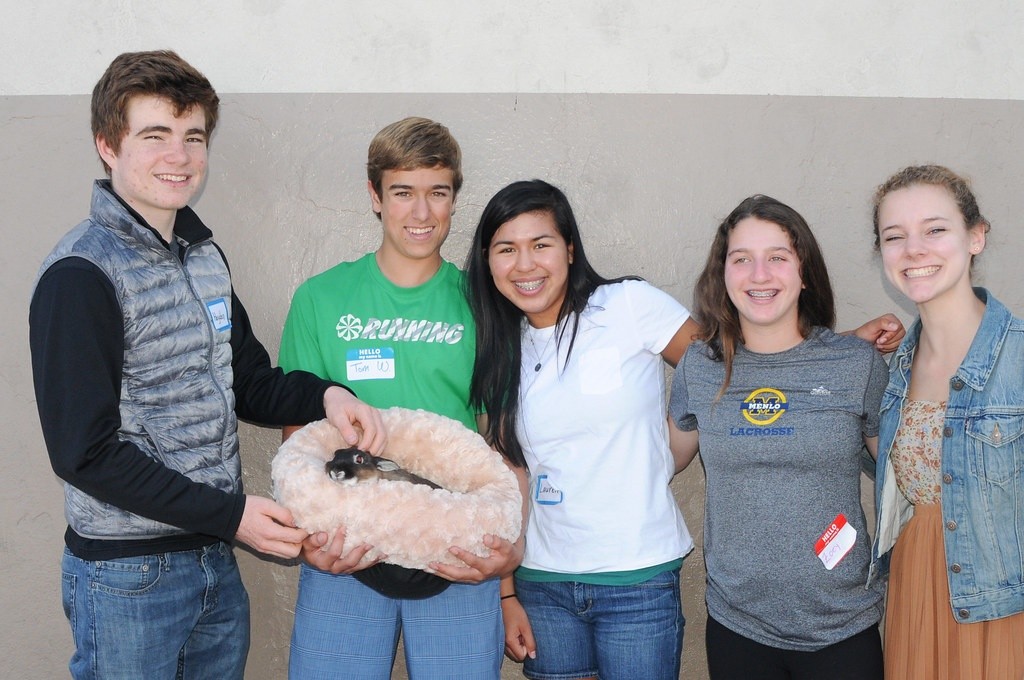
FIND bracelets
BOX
[500,594,517,601]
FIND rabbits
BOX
[323,444,455,491]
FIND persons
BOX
[29,48,385,680]
[667,195,889,680]
[460,179,906,680]
[277,116,530,680]
[865,164,1024,679]
